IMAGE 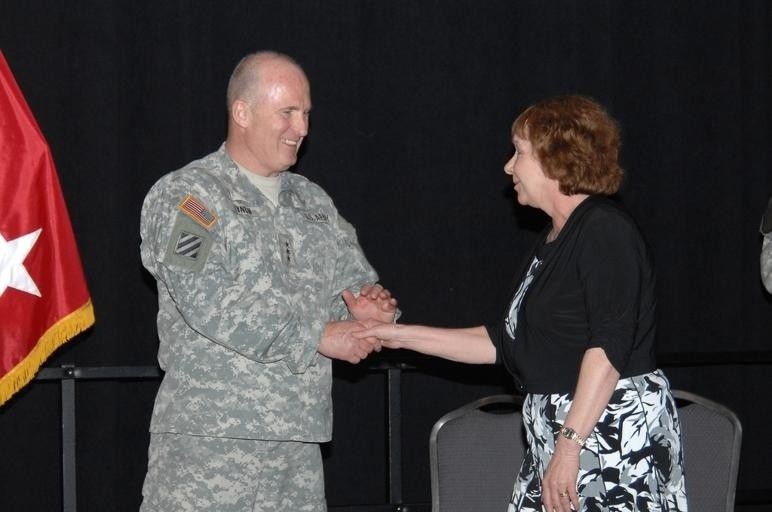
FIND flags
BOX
[0,49,96,403]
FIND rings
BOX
[559,491,568,497]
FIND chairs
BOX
[429,389,742,508]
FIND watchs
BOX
[556,427,587,447]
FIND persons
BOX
[349,91,694,511]
[133,49,405,511]
